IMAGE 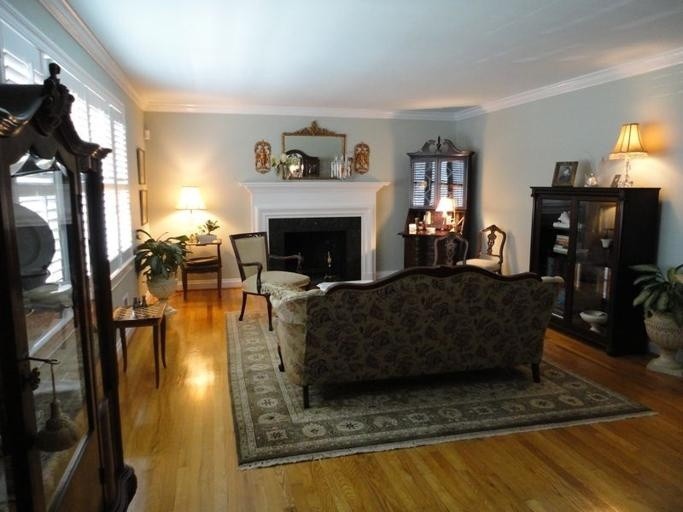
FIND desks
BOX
[112,301,167,389]
[177,239,222,301]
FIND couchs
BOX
[270,264,566,411]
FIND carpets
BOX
[223,308,658,472]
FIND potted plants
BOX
[629,263,683,380]
[133,228,193,300]
[195,219,219,245]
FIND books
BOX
[553,233,592,254]
[575,262,613,301]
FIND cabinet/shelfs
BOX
[285,149,320,177]
[528,185,662,357]
[0,61,138,512]
[396,135,474,269]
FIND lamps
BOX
[608,122,647,188]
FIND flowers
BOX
[270,153,298,172]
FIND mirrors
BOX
[282,120,346,179]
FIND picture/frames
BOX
[136,147,149,225]
[551,161,578,187]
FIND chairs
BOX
[432,224,506,276]
[228,231,312,332]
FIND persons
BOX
[558,166,572,182]
